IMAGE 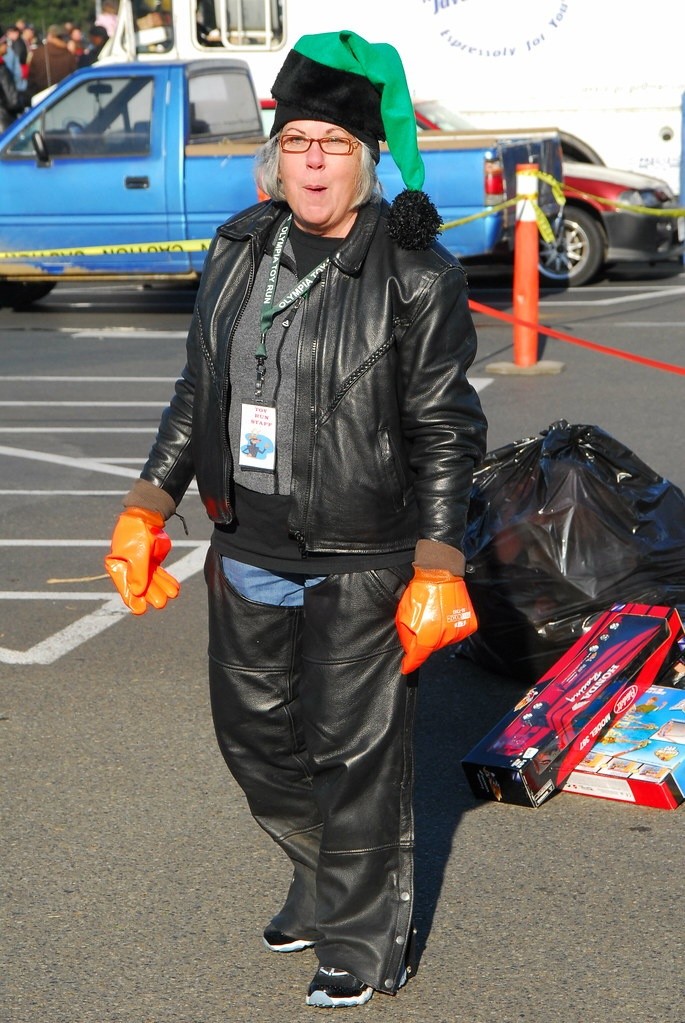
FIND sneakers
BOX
[305,967,407,1008]
[262,923,318,952]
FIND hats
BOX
[269,30,444,251]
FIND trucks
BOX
[16,0,685,203]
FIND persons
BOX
[105,30,489,1007]
[0,17,110,133]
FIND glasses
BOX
[276,135,360,155]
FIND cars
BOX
[192,92,685,290]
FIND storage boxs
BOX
[460,603,685,809]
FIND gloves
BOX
[104,507,181,616]
[394,563,478,675]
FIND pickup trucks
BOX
[0,55,563,308]
